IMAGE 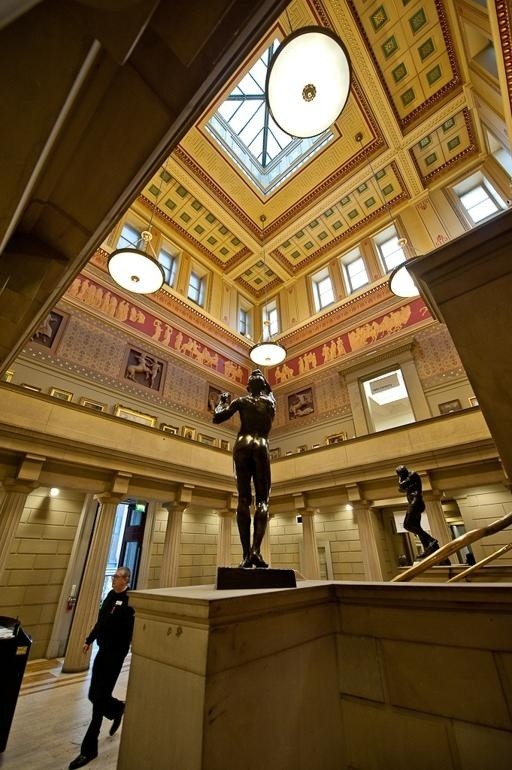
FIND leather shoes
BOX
[110,701,125,736]
[69,753,98,769]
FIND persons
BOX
[67,277,249,387]
[395,464,438,559]
[275,302,412,385]
[66,566,136,769]
[213,369,277,568]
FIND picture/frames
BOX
[78,395,110,415]
[46,385,74,404]
[160,421,181,438]
[437,398,460,414]
[112,402,158,429]
[468,397,478,406]
[215,437,231,451]
[197,432,219,449]
[268,430,348,459]
[181,424,198,441]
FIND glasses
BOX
[111,574,125,580]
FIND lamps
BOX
[265,29,351,139]
[106,161,175,294]
[249,215,288,367]
[48,488,59,499]
[362,143,432,298]
